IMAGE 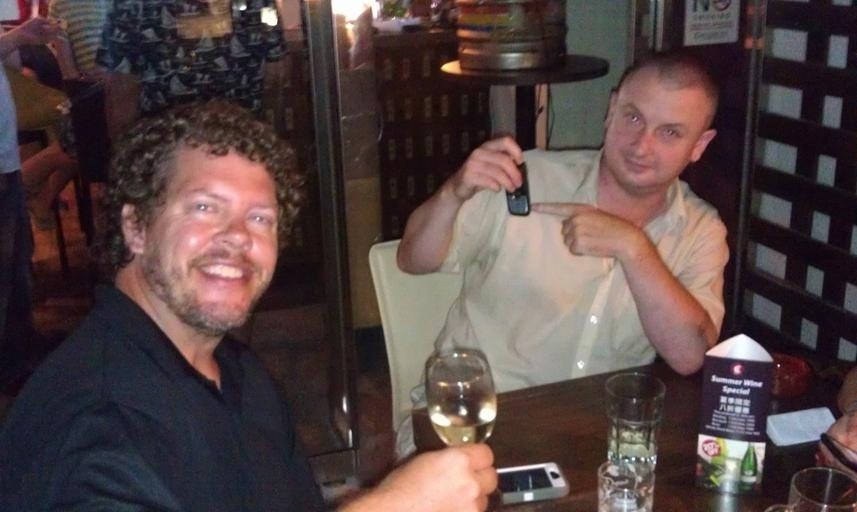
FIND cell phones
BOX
[505,161,531,216]
[820,433,857,473]
[496,462,570,505]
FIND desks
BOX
[408,342,853,509]
[438,51,609,150]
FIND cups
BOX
[597,373,667,512]
[764,467,856,511]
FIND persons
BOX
[47,1,131,165]
[813,415,852,476]
[2,27,75,229]
[0,1,68,396]
[1,100,501,512]
[395,57,731,463]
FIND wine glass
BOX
[424,345,502,447]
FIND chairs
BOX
[366,229,509,436]
